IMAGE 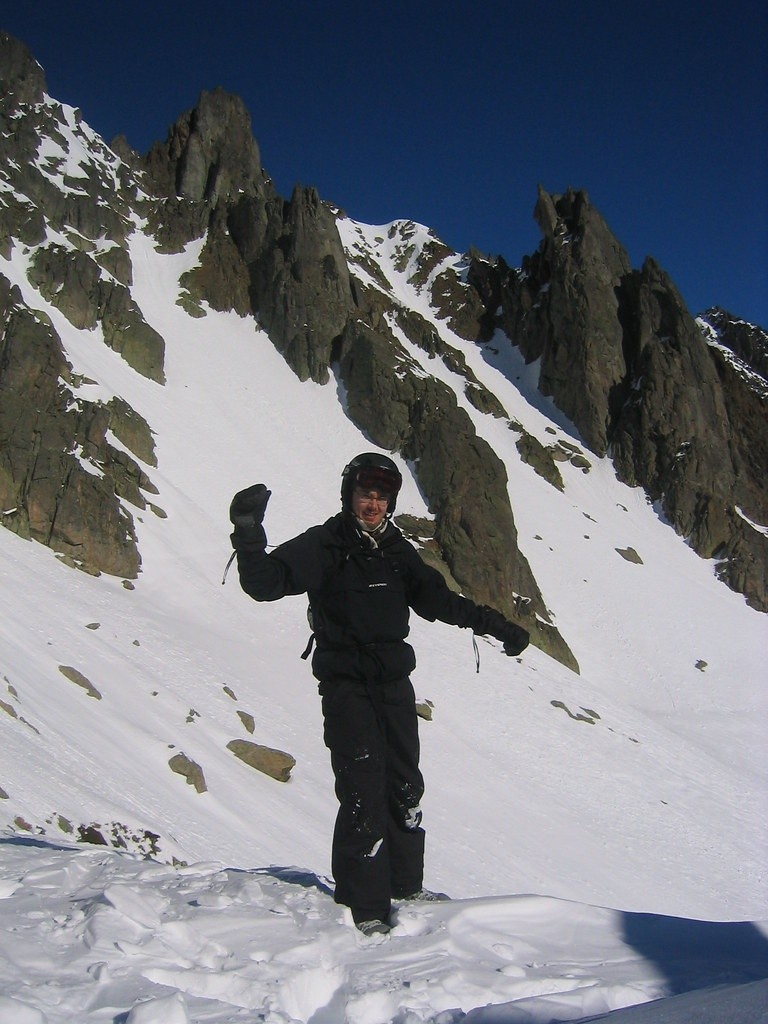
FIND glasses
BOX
[353,488,390,506]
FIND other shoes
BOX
[355,919,391,940]
[401,888,450,903]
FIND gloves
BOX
[218,484,280,584]
[472,606,531,673]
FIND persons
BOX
[229,452,532,935]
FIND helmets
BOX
[340,453,402,513]
